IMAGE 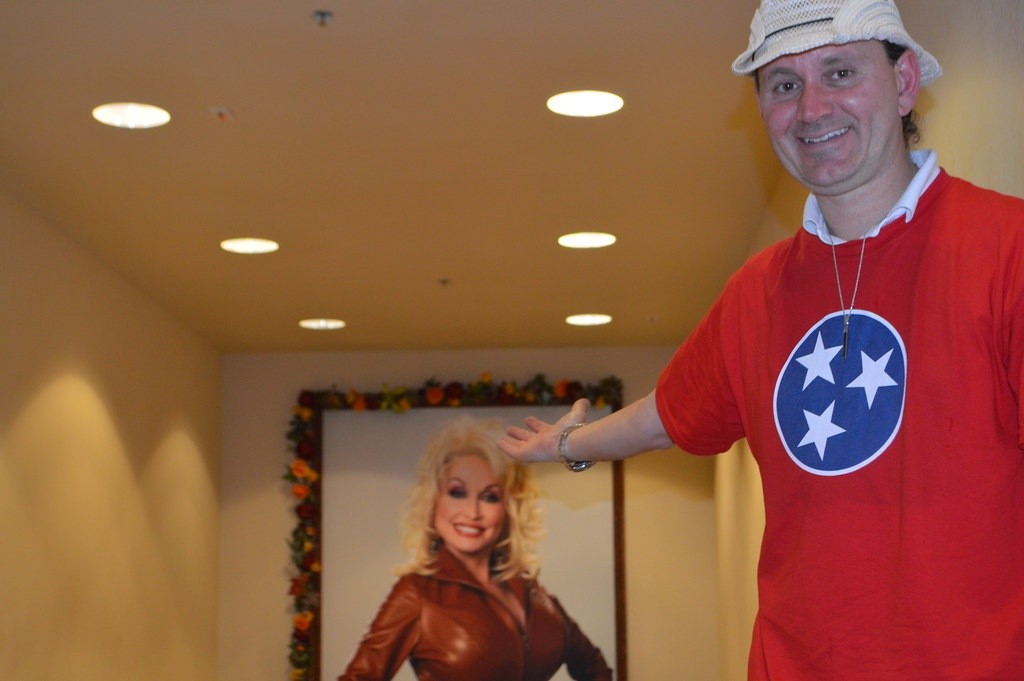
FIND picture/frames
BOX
[287,376,628,680]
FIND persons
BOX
[337,421,613,681]
[495,0,1024,681]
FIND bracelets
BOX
[558,423,597,472]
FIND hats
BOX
[730,0,941,87]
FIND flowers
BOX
[286,377,622,681]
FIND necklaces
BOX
[829,233,865,359]
